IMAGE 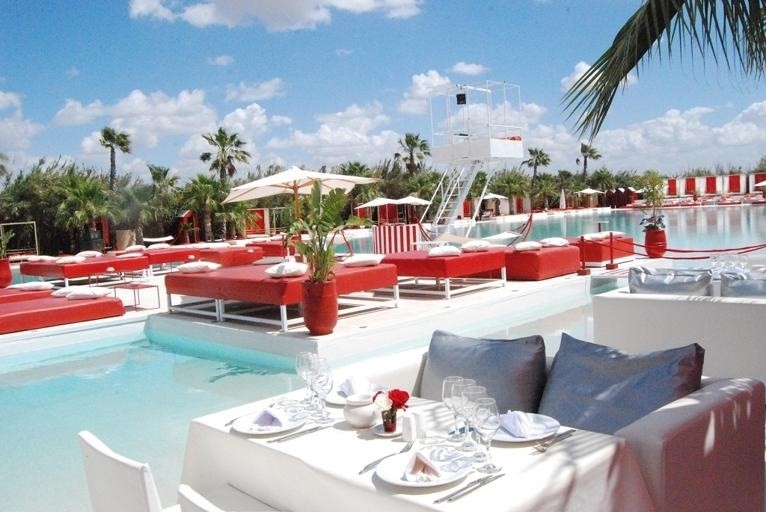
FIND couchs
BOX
[409,353,764,512]
[590,286,765,384]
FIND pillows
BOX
[629,264,764,295]
[418,329,705,434]
[575,230,626,242]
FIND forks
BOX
[534,428,576,452]
[225,400,283,426]
[359,441,414,474]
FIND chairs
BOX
[76,430,276,512]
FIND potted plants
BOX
[283,178,374,337]
[0,228,16,288]
[636,169,667,258]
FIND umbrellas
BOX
[754,179,766,189]
[558,188,567,211]
[218,164,390,263]
[352,197,398,224]
[478,191,509,201]
[574,189,605,208]
[390,196,433,224]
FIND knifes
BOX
[435,472,505,502]
[266,425,330,443]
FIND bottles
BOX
[402,412,426,443]
[344,393,382,428]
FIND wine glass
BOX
[294,350,334,424]
[442,376,501,473]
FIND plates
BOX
[326,384,372,404]
[375,451,473,487]
[371,423,402,437]
[233,409,306,436]
[493,411,561,442]
